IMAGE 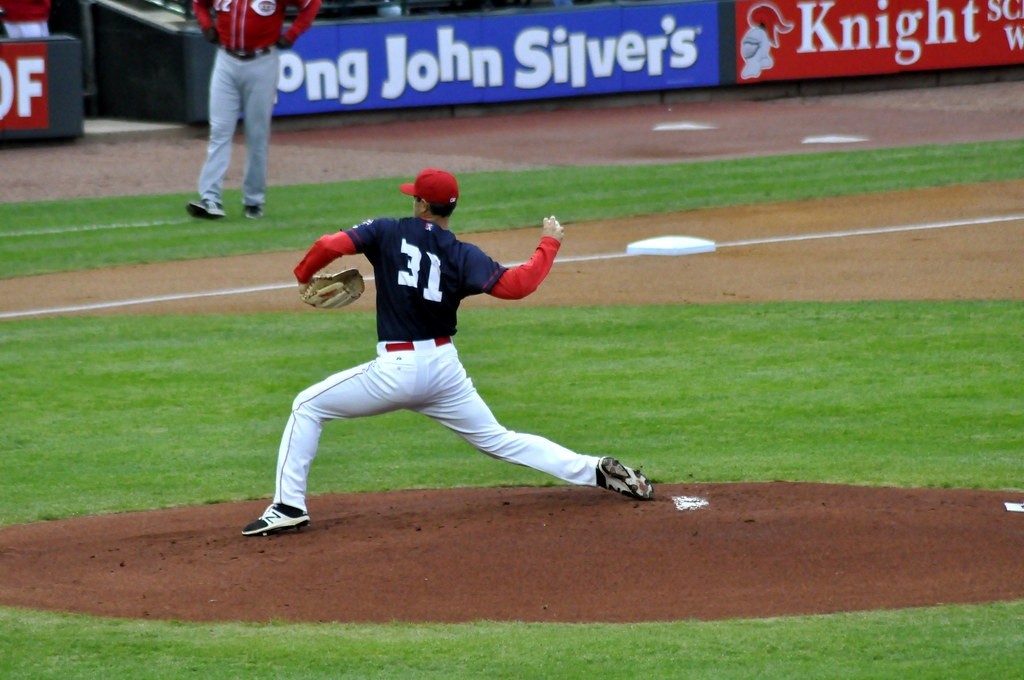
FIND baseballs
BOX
[548,220,560,228]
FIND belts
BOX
[384,337,452,352]
[222,45,278,61]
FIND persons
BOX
[0,0,50,37]
[183,0,320,220]
[241,167,656,536]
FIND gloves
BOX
[274,34,292,51]
[204,27,218,44]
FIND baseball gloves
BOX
[297,265,365,310]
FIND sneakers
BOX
[595,457,655,500]
[242,206,264,219]
[241,503,309,537]
[185,200,225,220]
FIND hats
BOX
[400,167,459,203]
